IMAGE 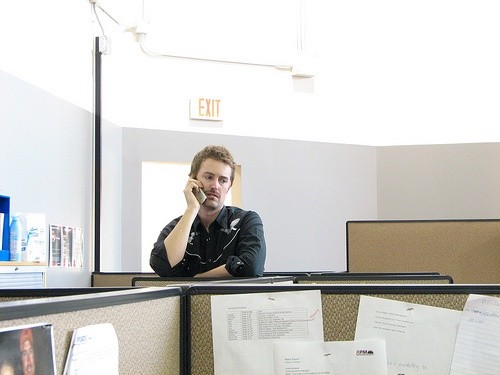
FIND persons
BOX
[149,145,266,280]
[0,329,36,375]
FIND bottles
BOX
[10,213,21,261]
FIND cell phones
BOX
[192,187,207,204]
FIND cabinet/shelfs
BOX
[0,261,47,289]
